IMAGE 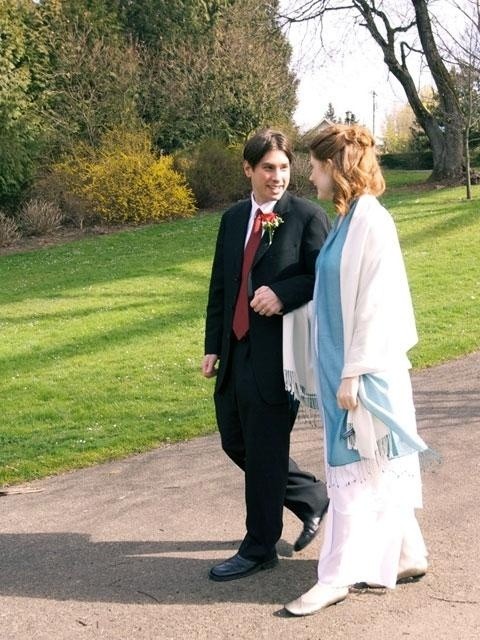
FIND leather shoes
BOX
[284,581,349,615]
[294,499,330,551]
[364,555,428,588]
[209,552,278,581]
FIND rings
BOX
[262,309,267,313]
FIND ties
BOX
[233,210,262,341]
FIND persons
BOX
[202,128,331,582]
[273,123,433,617]
